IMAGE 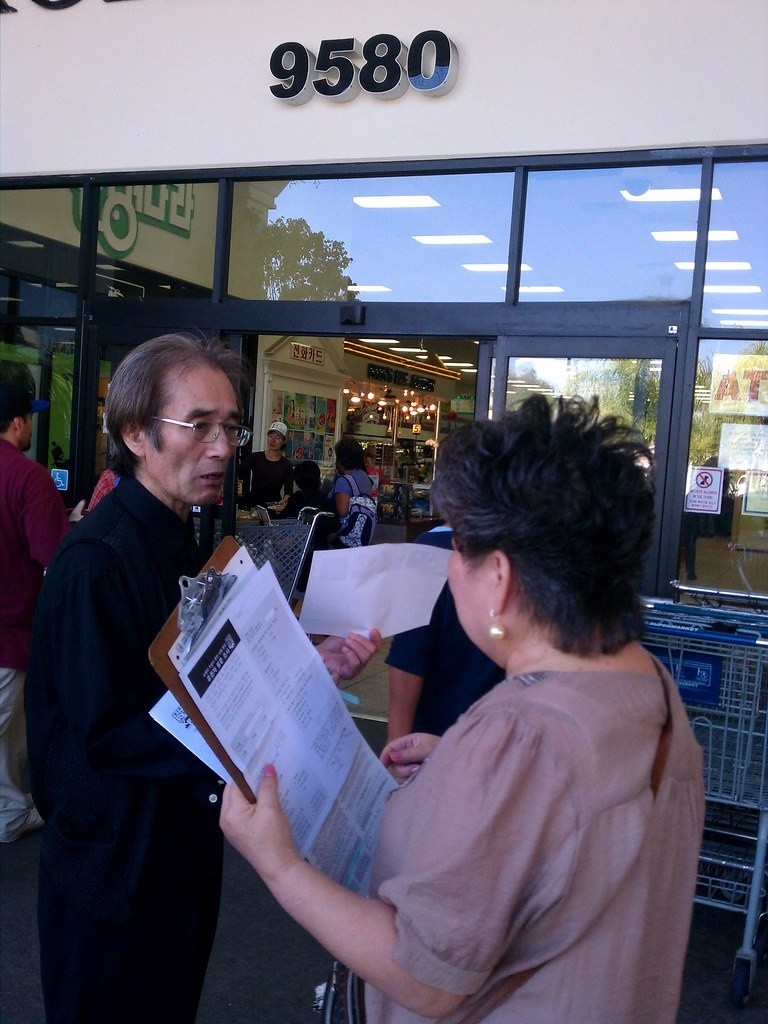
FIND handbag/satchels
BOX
[312,961,364,1024]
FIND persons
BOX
[86,420,386,546]
[24,333,384,1024]
[678,508,698,579]
[215,392,705,1024]
[0,359,89,843]
[383,518,507,749]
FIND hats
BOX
[268,421,288,440]
[30,398,50,413]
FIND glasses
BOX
[145,416,254,447]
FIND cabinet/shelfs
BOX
[343,434,434,524]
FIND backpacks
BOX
[335,473,378,547]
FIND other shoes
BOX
[0,808,46,843]
[23,793,33,806]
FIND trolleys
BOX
[191,507,335,607]
[632,581,768,1009]
